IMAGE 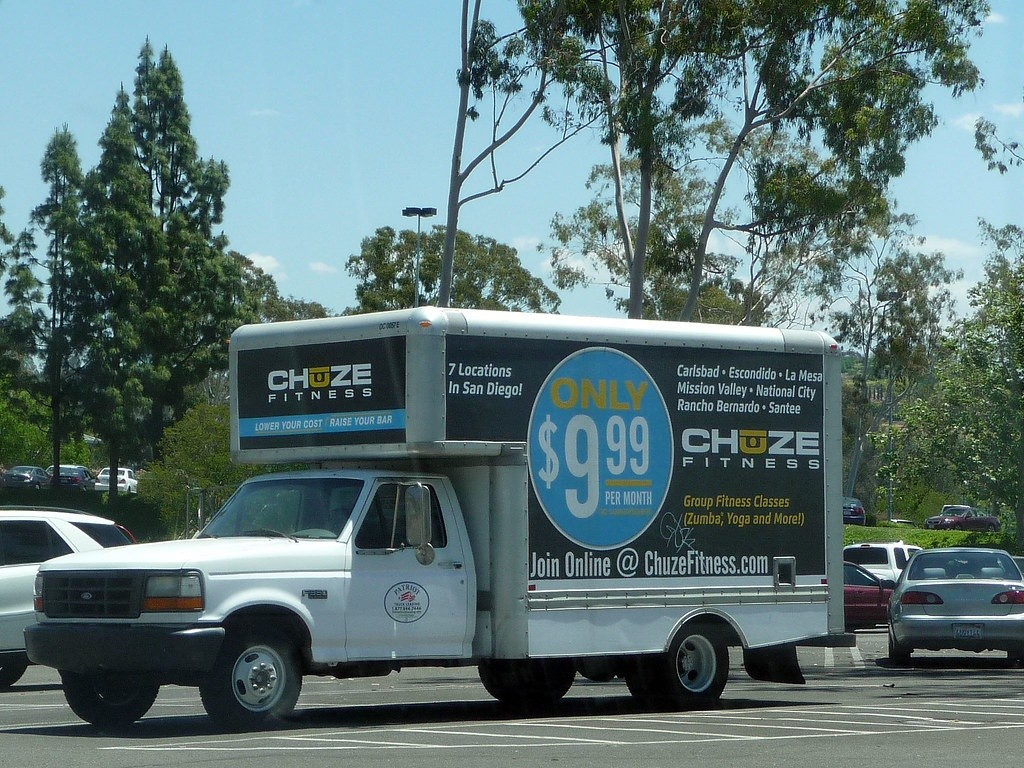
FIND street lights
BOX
[402,207,436,306]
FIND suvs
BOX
[0,506,136,688]
[842,539,1024,666]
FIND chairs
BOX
[980,567,1004,579]
[923,567,948,578]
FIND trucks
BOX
[25,307,845,735]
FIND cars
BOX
[924,504,1003,532]
[94,466,139,494]
[46,465,97,491]
[1,465,51,490]
[843,498,866,526]
